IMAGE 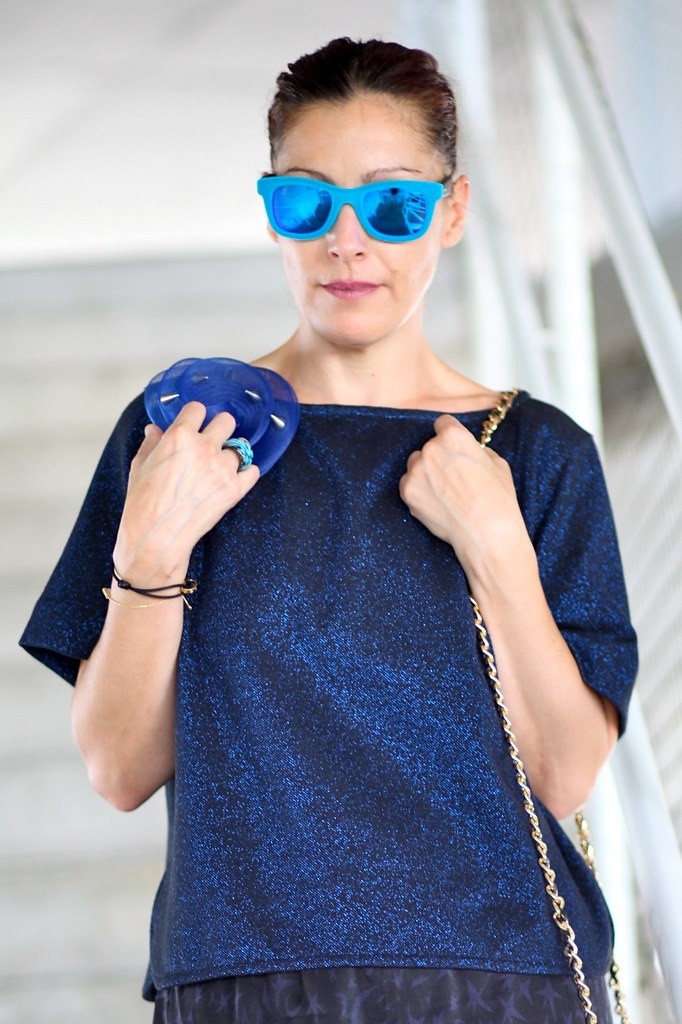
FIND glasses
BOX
[257,174,454,243]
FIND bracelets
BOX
[102,559,199,610]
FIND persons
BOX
[19,36,639,1024]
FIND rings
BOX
[222,437,254,471]
